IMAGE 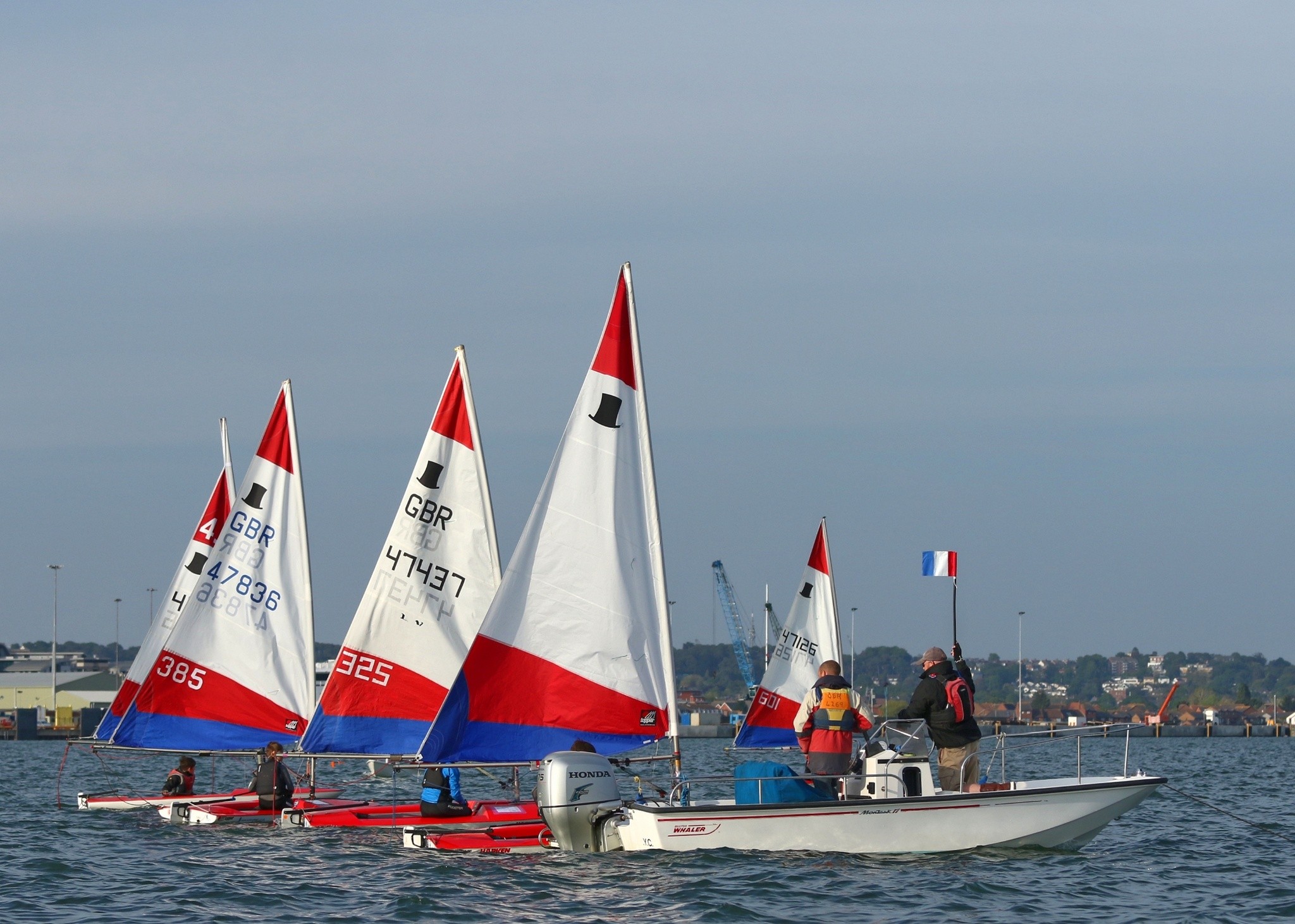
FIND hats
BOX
[179,756,196,772]
[911,646,947,665]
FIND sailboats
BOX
[56,258,1169,857]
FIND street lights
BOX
[147,587,159,625]
[46,563,65,724]
[850,606,859,689]
[1018,611,1026,720]
[113,598,122,690]
[669,601,676,626]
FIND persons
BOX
[898,640,982,793]
[162,757,196,796]
[420,760,472,817]
[256,741,295,811]
[793,659,876,799]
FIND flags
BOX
[921,551,957,578]
